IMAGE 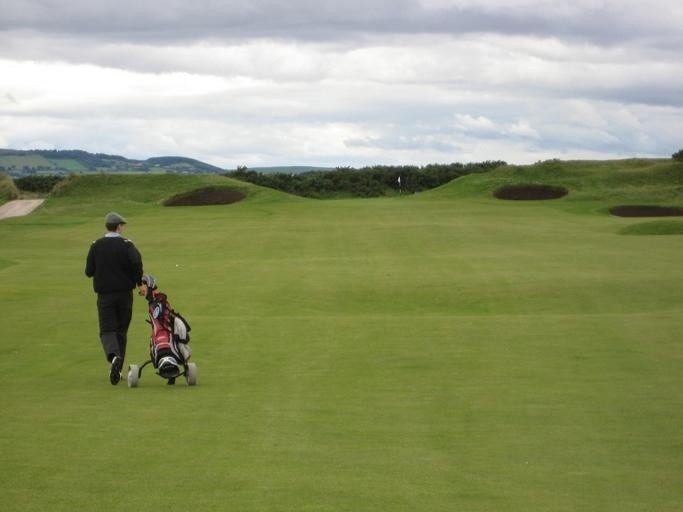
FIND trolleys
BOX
[125,273,196,387]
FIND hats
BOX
[104,212,130,224]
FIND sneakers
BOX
[111,356,121,385]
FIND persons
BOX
[85,212,146,385]
